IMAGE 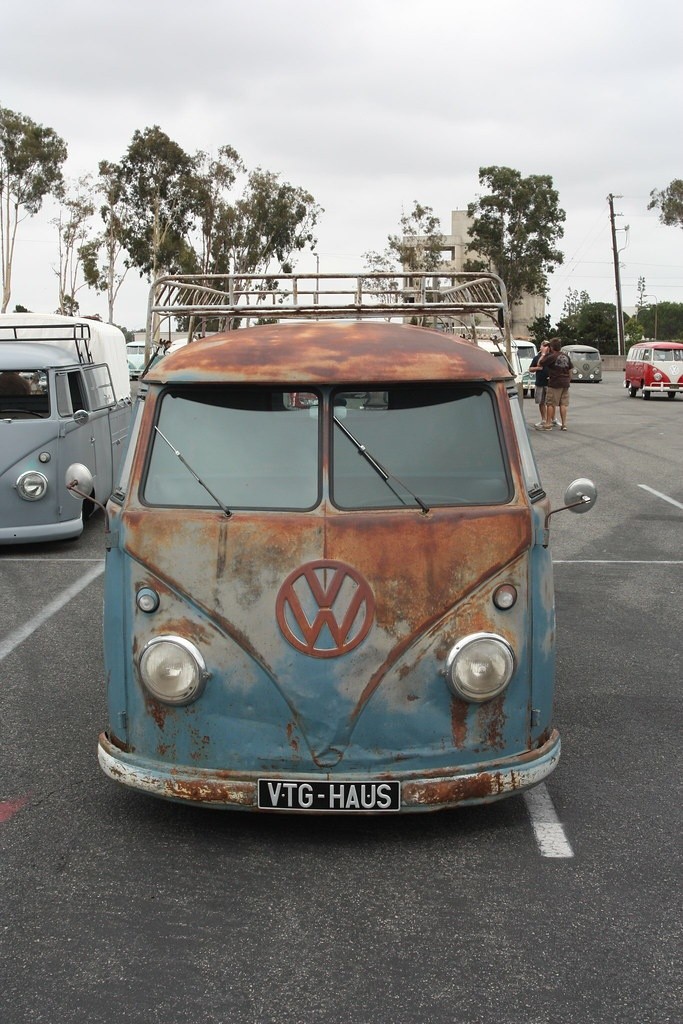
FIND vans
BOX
[472,338,538,399]
[625,341,683,400]
[123,339,163,383]
[0,311,134,545]
[560,343,604,383]
[63,270,597,813]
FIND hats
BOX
[541,340,551,345]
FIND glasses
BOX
[542,345,550,348]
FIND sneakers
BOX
[536,424,552,430]
[551,418,562,427]
[561,425,567,431]
[535,420,546,427]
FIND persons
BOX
[529,337,574,431]
